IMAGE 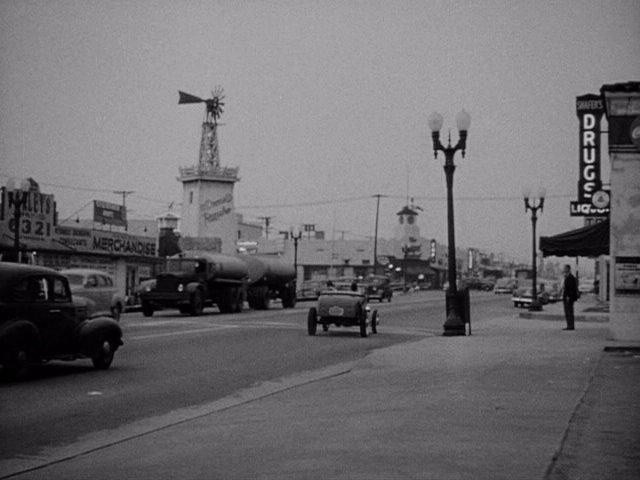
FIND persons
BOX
[351,280,359,293]
[561,264,580,331]
[327,280,338,292]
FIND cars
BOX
[494,278,563,308]
[0,261,124,373]
[458,277,495,292]
[298,275,393,338]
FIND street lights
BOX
[522,186,547,311]
[428,108,472,336]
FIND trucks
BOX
[135,248,297,317]
